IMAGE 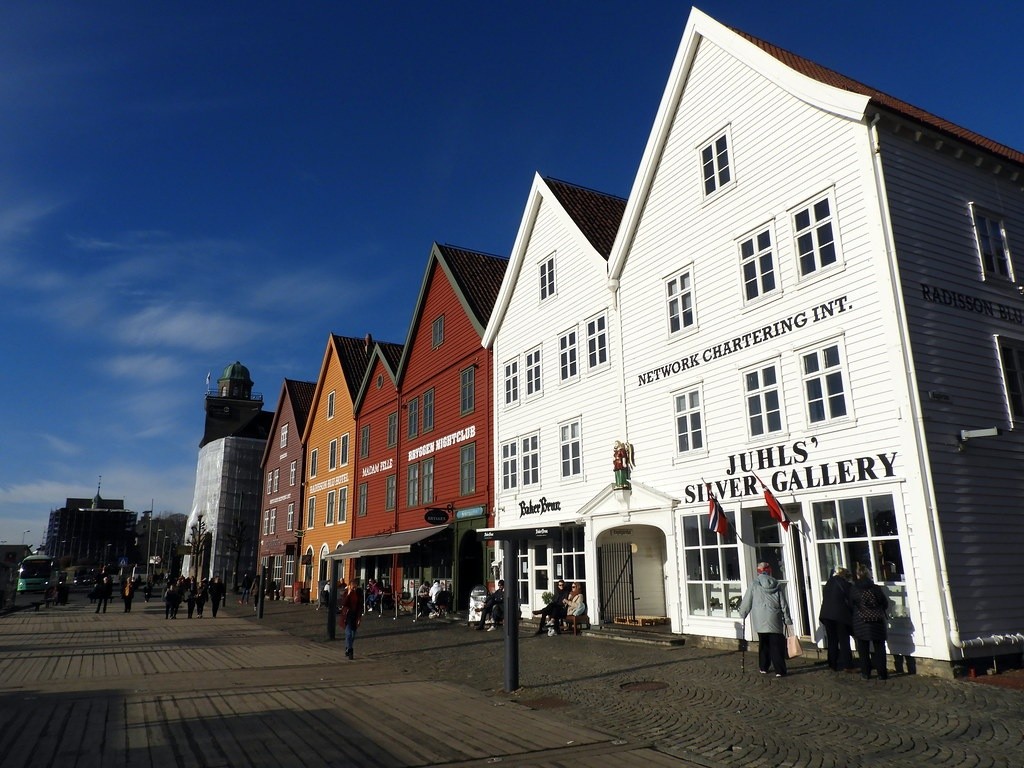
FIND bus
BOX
[64,566,91,585]
[102,564,131,583]
[131,564,160,584]
[16,555,58,595]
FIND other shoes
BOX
[536,629,544,635]
[254,607,256,611]
[775,673,781,676]
[548,629,557,637]
[345,646,353,660]
[165,615,216,619]
[475,607,483,612]
[486,625,497,631]
[532,610,541,615]
[545,621,555,626]
[475,625,482,630]
[862,673,869,679]
[759,670,767,673]
[239,600,243,605]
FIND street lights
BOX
[22,530,30,545]
[106,543,112,563]
[154,528,164,573]
[163,535,171,571]
[62,540,67,556]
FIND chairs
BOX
[432,591,451,617]
[376,586,396,613]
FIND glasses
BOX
[559,584,564,586]
[572,586,576,588]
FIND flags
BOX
[762,483,790,531]
[706,485,729,536]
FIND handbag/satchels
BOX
[783,625,803,659]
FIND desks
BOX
[418,595,432,617]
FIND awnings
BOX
[325,527,447,561]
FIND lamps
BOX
[302,481,309,486]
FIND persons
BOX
[417,579,443,618]
[47,571,225,620]
[250,575,260,611]
[341,580,365,656]
[738,562,793,677]
[820,567,861,673]
[365,579,381,612]
[323,578,348,608]
[849,564,890,680]
[474,579,504,631]
[533,580,583,636]
[238,573,253,604]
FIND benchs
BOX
[547,615,590,636]
